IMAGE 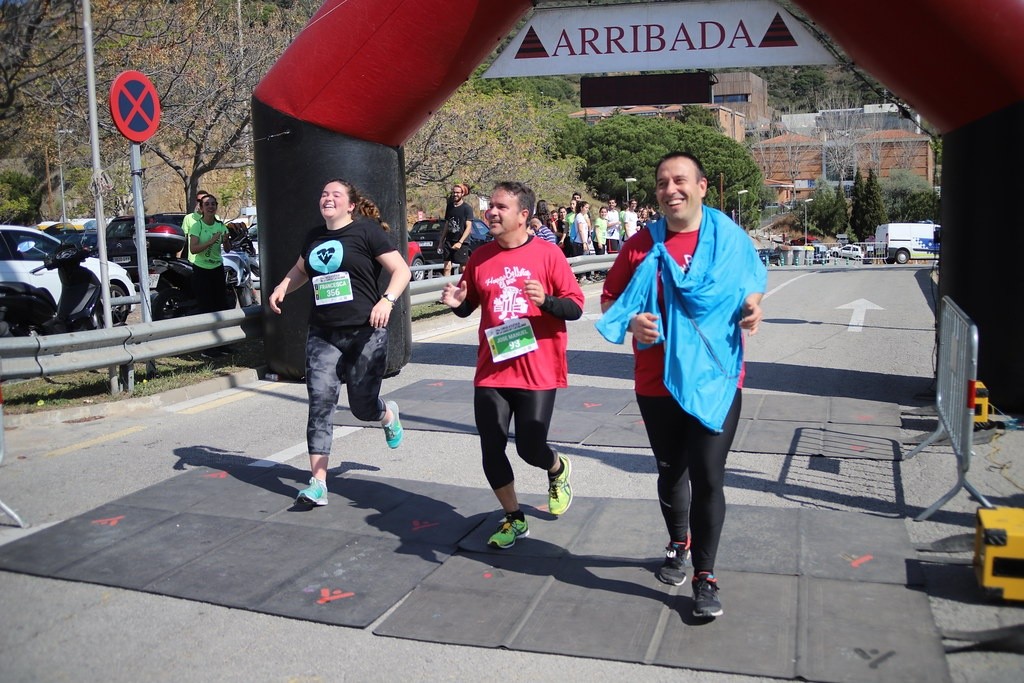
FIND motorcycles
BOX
[132,222,258,322]
[0,220,127,338]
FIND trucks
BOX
[875,223,941,264]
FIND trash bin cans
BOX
[782,246,793,265]
[793,246,805,266]
[805,246,815,266]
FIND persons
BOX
[440,181,585,550]
[436,183,474,276]
[594,151,767,617]
[269,178,412,507]
[528,192,663,284]
[177,190,232,359]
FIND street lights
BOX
[738,190,749,226]
[804,199,813,245]
[626,178,637,203]
[57,130,73,225]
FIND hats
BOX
[457,184,469,197]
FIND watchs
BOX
[383,294,397,303]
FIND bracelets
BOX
[459,242,462,244]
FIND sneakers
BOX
[547,456,573,515]
[691,574,723,617]
[658,534,689,586]
[487,518,529,549]
[382,401,402,449]
[298,476,328,505]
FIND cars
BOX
[790,237,820,246]
[0,225,137,324]
[34,212,189,283]
[409,219,494,278]
[220,224,261,290]
[757,249,781,268]
[407,232,426,282]
[812,245,830,264]
[831,245,864,261]
